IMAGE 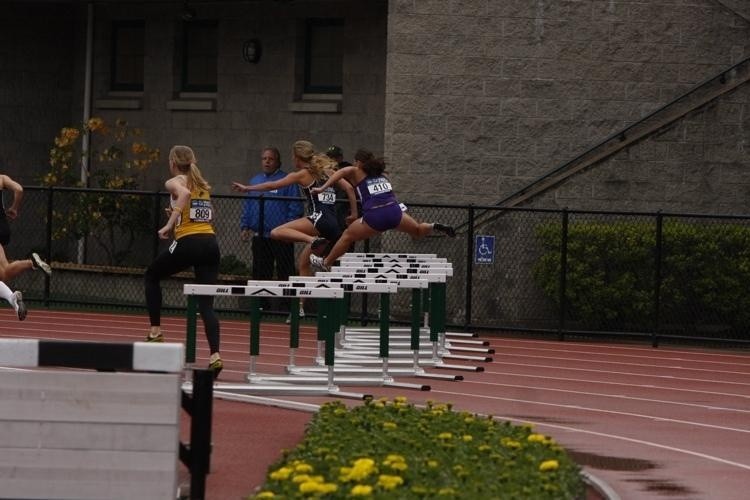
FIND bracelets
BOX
[173,206,184,213]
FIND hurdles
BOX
[339,252,496,355]
[330,266,494,362]
[0,335,212,500]
[285,275,466,382]
[180,283,374,413]
[314,271,484,374]
[246,279,433,391]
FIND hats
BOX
[325,143,343,156]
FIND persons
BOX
[0,174,52,321]
[309,146,456,272]
[143,145,223,380]
[322,145,358,219]
[240,146,304,310]
[231,141,359,324]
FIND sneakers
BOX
[430,222,455,239]
[28,252,51,276]
[285,308,305,324]
[142,333,165,343]
[12,290,27,321]
[309,238,331,271]
[208,359,223,379]
